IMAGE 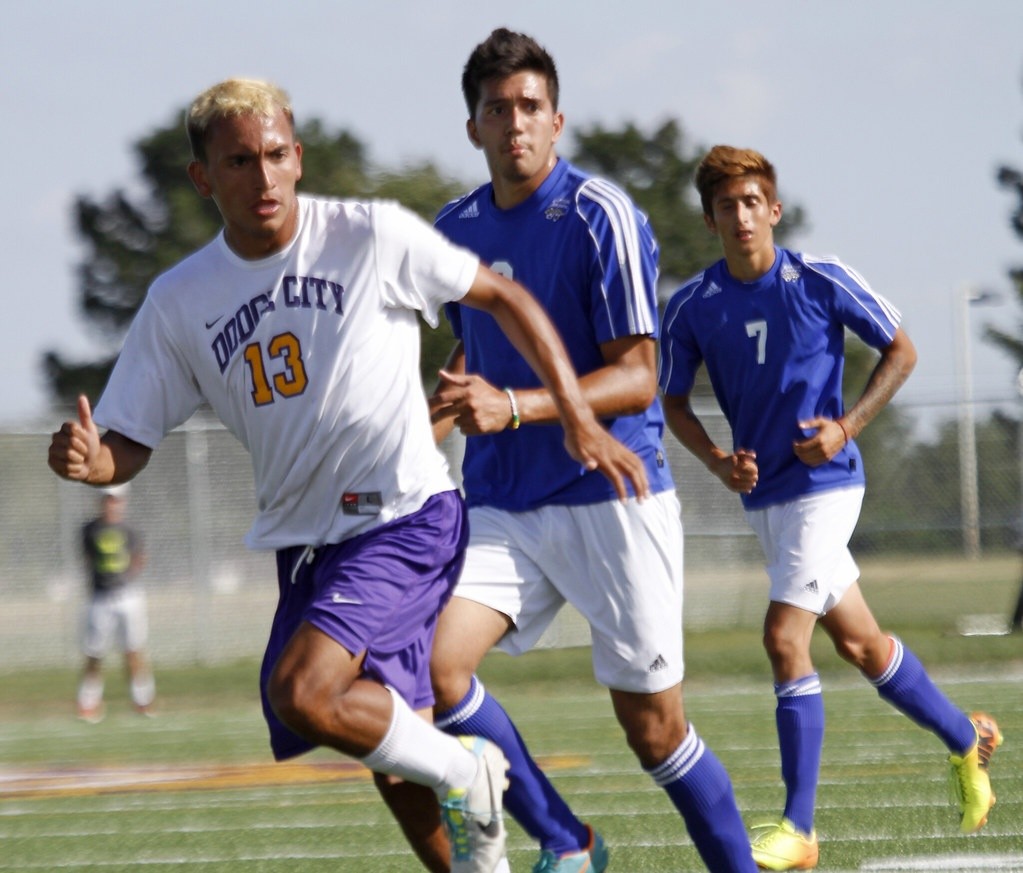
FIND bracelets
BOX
[503,388,520,430]
[836,420,850,443]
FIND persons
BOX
[49,79,651,873]
[657,146,1004,871]
[77,484,157,724]
[427,28,755,873]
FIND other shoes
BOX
[135,701,158,719]
[75,697,108,727]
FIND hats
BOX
[102,483,129,496]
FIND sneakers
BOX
[436,735,511,873]
[751,816,818,871]
[945,710,1004,834]
[531,822,610,873]
